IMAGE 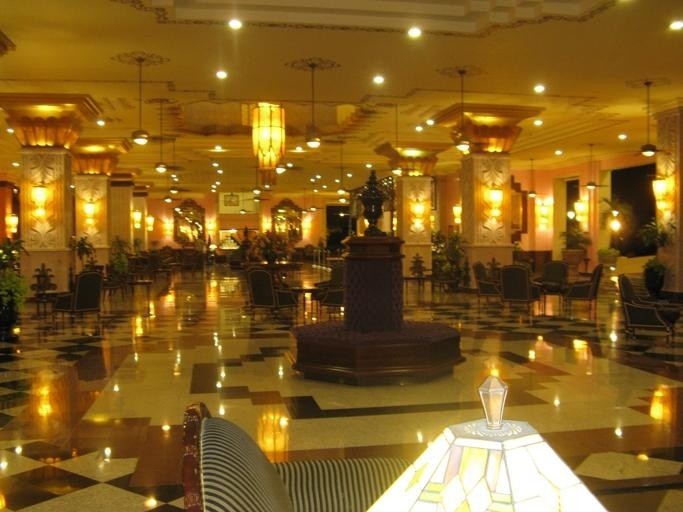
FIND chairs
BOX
[469,257,603,328]
[52,245,205,324]
[617,273,681,344]
[178,396,413,511]
[245,259,345,319]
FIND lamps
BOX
[374,96,547,175]
[5,90,154,198]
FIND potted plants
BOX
[643,259,666,297]
[557,220,592,268]
